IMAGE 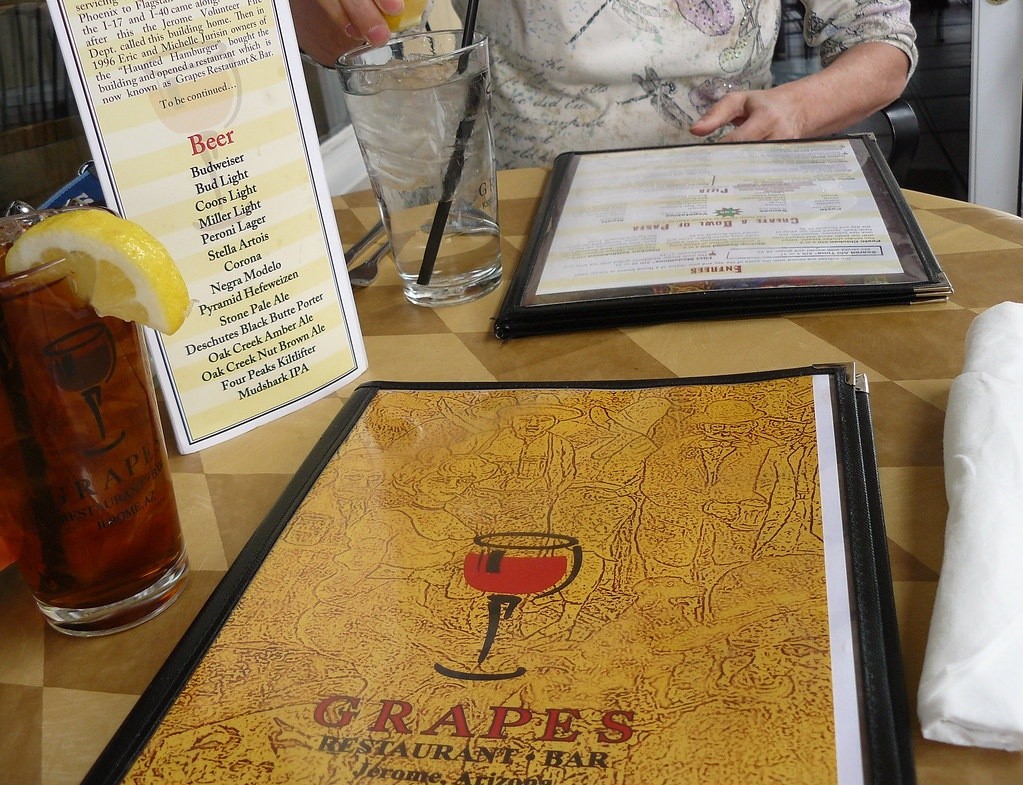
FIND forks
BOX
[348,242,391,287]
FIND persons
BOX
[289,0,919,171]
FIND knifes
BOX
[344,218,385,267]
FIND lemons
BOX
[381,0,428,33]
[5,209,189,336]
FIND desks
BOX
[0,168,1023,785]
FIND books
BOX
[77,361,919,785]
[491,129,954,336]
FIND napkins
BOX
[916,299,1023,754]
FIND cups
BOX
[0,208,189,638]
[337,30,504,308]
